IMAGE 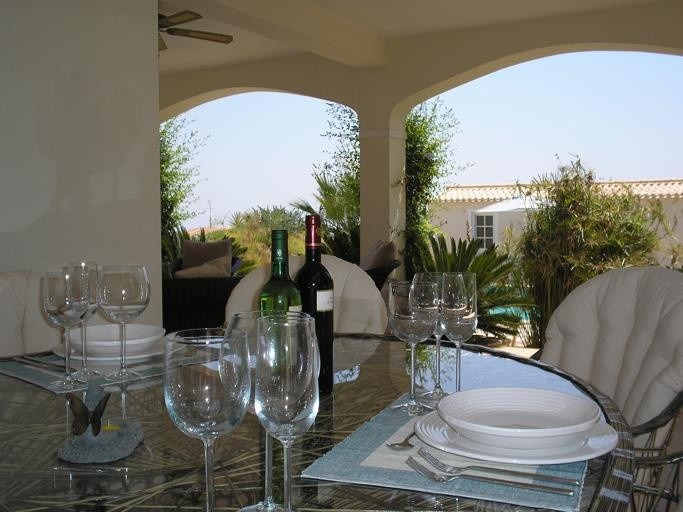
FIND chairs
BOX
[352,260,403,296]
[223,254,388,335]
[0,270,103,359]
[169,254,241,277]
[533,265,682,478]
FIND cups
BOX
[47,392,156,500]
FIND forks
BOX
[400,455,576,500]
[414,444,584,489]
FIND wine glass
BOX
[386,267,479,416]
[37,258,168,389]
[160,308,320,510]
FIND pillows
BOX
[181,238,233,269]
[366,238,395,265]
[173,254,232,277]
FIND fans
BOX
[159,10,231,52]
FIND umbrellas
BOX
[477,195,557,216]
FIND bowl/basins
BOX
[435,386,603,444]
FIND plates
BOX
[413,404,619,467]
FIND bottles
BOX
[299,389,341,507]
[255,229,301,382]
[293,213,334,392]
